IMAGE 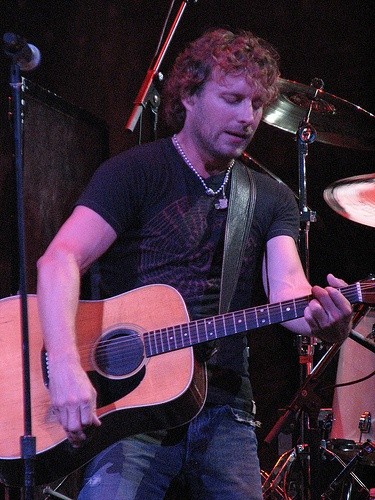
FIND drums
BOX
[306,307,375,463]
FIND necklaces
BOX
[173,133,235,209]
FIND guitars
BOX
[0,272,375,488]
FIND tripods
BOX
[262,121,375,500]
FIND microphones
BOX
[2,31,40,71]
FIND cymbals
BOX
[262,76,375,152]
[322,172,375,230]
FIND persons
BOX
[36,27,353,500]
